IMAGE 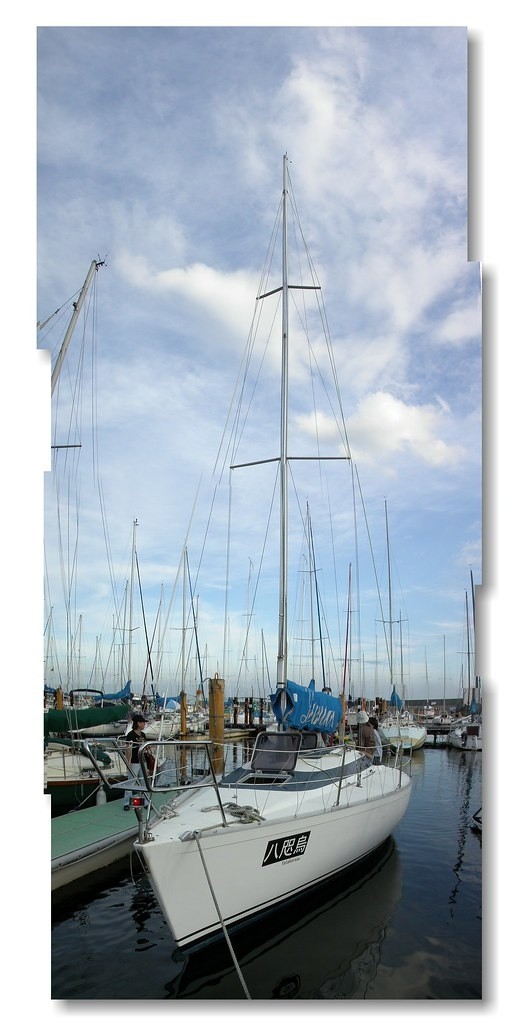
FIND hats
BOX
[132,715,149,722]
[356,711,369,723]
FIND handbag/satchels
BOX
[144,748,155,770]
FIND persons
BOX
[356,711,376,758]
[367,716,383,765]
[124,715,155,811]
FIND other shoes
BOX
[145,805,153,810]
[124,805,130,810]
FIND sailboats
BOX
[162,834,401,999]
[36,155,483,957]
